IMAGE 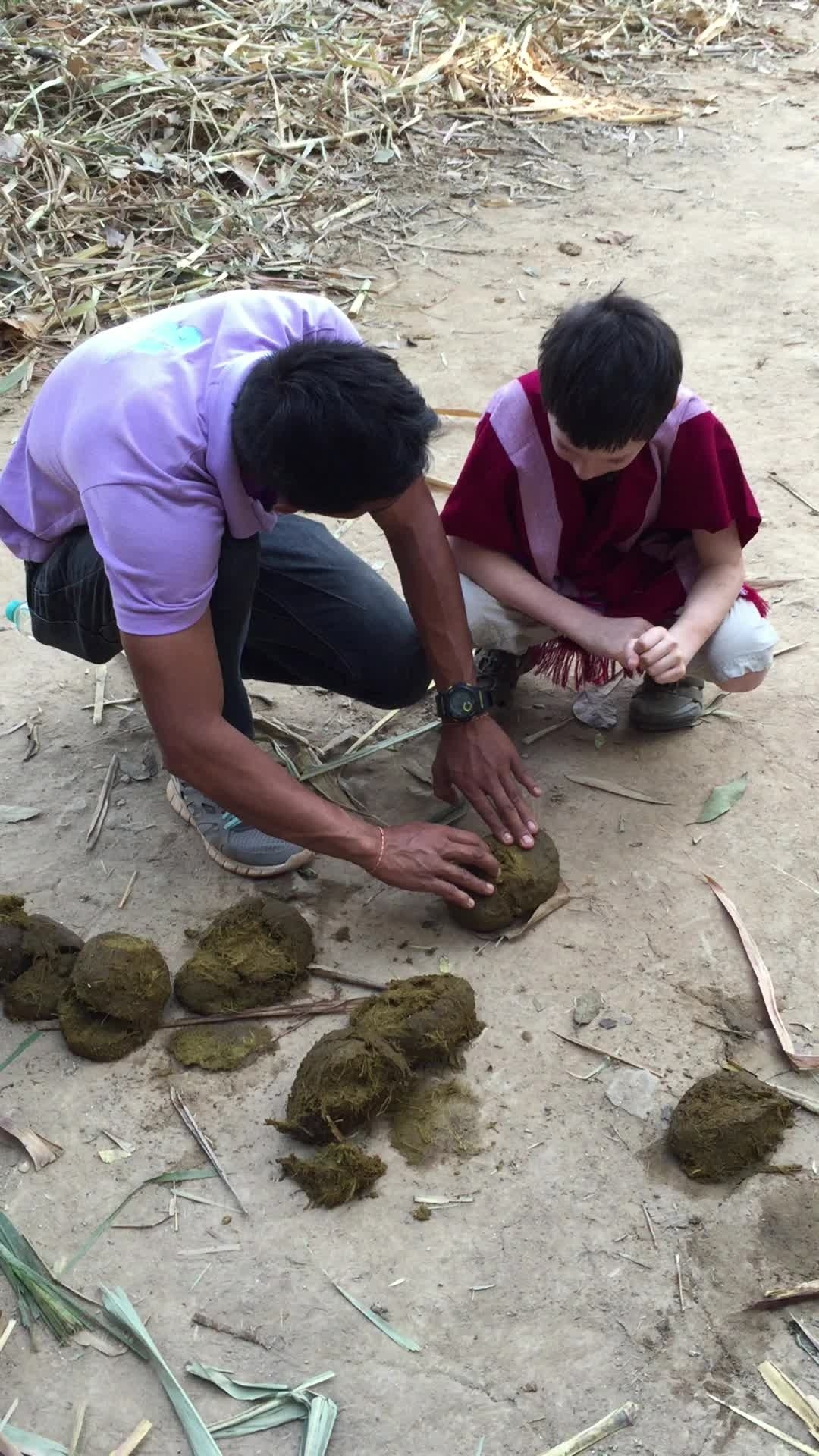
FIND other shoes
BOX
[473,647,545,723]
[630,672,703,733]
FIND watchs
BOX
[435,681,487,724]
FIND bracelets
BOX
[369,825,384,873]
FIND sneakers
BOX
[166,777,315,875]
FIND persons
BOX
[439,277,779,732]
[0,289,542,909]
